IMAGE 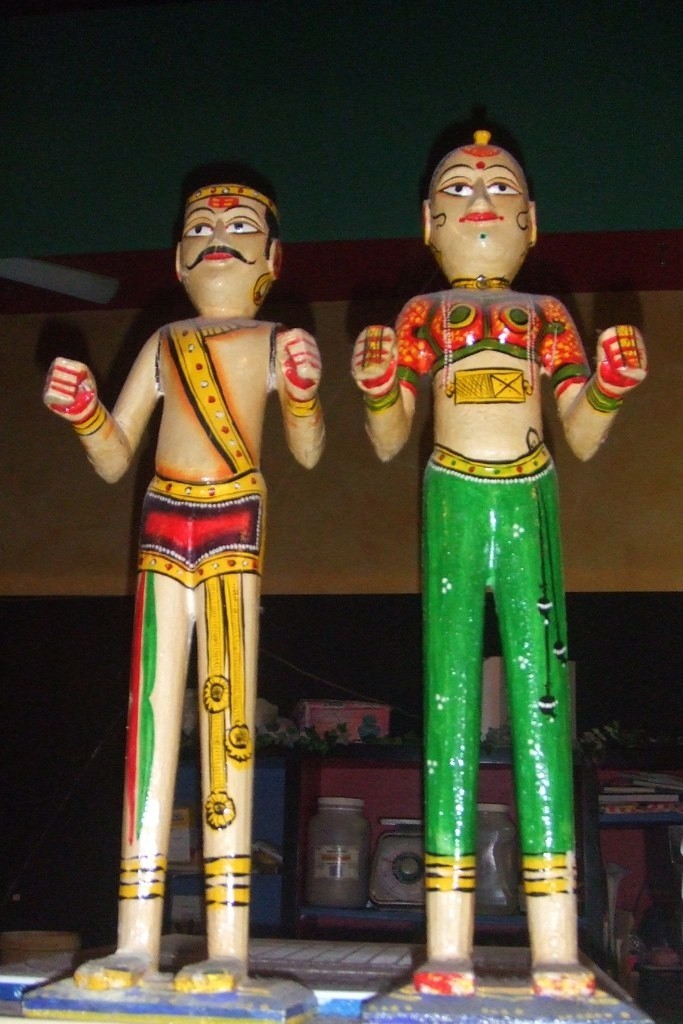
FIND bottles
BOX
[476,801,516,916]
[304,794,372,906]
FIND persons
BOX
[43,186,325,996]
[354,130,647,1000]
